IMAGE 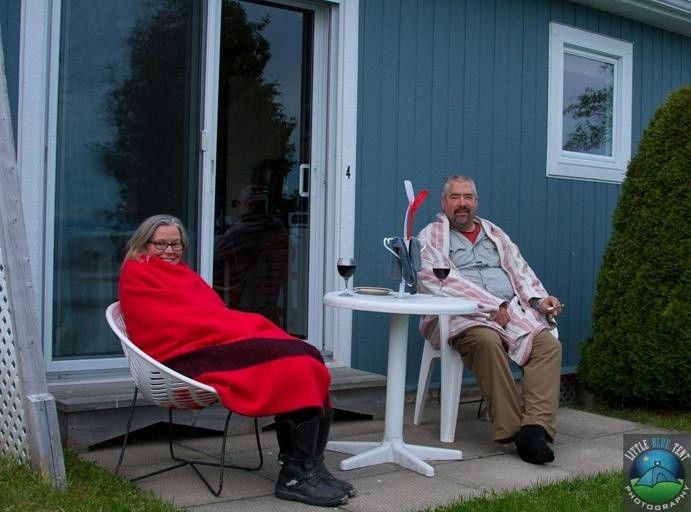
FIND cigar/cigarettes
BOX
[548,303,566,311]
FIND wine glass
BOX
[432,259,452,297]
[336,257,357,298]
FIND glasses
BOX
[147,238,184,251]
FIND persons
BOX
[412,171,569,464]
[116,213,358,507]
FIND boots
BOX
[274,409,356,507]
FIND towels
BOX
[387,234,421,292]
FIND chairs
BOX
[411,313,559,445]
[102,298,263,499]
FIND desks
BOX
[319,288,481,480]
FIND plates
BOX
[353,286,392,295]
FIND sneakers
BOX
[514,431,555,465]
